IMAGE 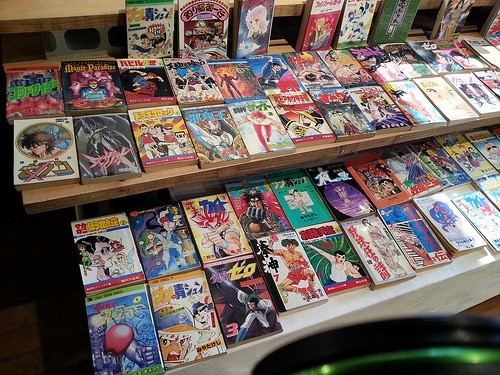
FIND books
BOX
[125,0,174,59]
[429,0,476,40]
[295,0,344,53]
[177,0,230,60]
[480,0,500,38]
[332,0,378,50]
[68,124,500,375]
[367,0,422,48]
[5,37,500,192]
[230,0,275,59]
